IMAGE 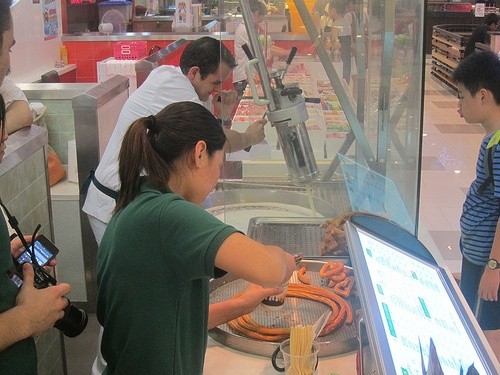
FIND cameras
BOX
[6,261,88,338]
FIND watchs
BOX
[486,256,500,269]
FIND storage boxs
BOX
[98,1,132,25]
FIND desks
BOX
[50,164,88,303]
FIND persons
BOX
[0,0,72,375]
[80,36,267,375]
[464,13,498,59]
[209,0,370,100]
[97,102,296,375]
[453,50,500,363]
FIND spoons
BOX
[286,324,313,375]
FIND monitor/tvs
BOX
[344,220,500,375]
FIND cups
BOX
[280,339,318,375]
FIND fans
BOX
[98,8,126,36]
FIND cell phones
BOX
[16,234,60,268]
